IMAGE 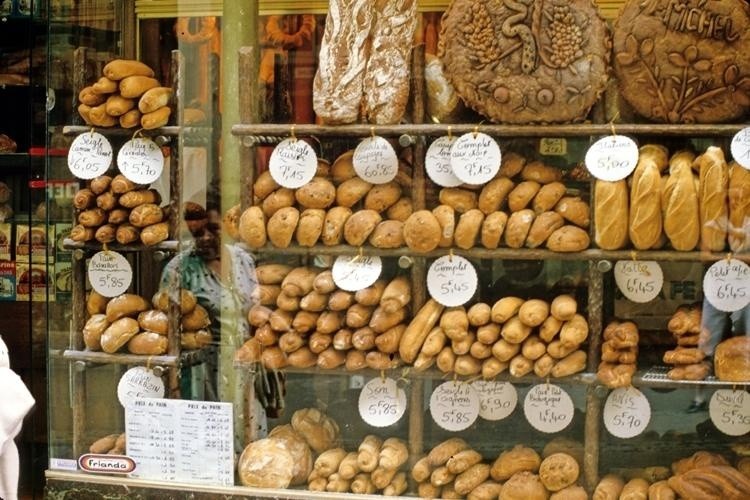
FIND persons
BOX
[158,177,271,471]
[0,331,34,500]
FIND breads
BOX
[248,304,270,327]
[105,98,134,115]
[592,475,625,497]
[606,322,640,343]
[180,331,211,347]
[311,335,332,355]
[120,191,158,207]
[495,343,518,362]
[90,177,109,193]
[338,177,372,205]
[313,477,326,489]
[120,76,163,96]
[289,347,312,366]
[443,310,470,342]
[255,265,288,283]
[504,210,534,248]
[365,183,399,211]
[455,209,483,248]
[440,351,453,370]
[526,212,560,249]
[446,487,466,495]
[715,336,749,384]
[262,189,293,216]
[539,458,580,489]
[453,339,470,354]
[491,444,538,478]
[314,159,327,177]
[375,326,402,354]
[367,308,405,332]
[370,469,391,485]
[328,291,353,310]
[697,146,727,250]
[290,314,315,329]
[277,292,304,312]
[388,200,410,219]
[510,182,537,211]
[68,227,91,244]
[422,329,445,358]
[671,368,710,381]
[272,313,295,330]
[97,195,116,210]
[426,437,465,456]
[280,333,302,353]
[87,287,105,314]
[629,142,666,252]
[294,180,333,206]
[550,295,577,321]
[298,291,327,312]
[535,356,558,377]
[93,78,117,95]
[550,343,571,358]
[552,352,591,377]
[181,305,209,328]
[470,302,488,325]
[255,329,275,346]
[236,339,259,365]
[661,347,708,363]
[730,151,748,252]
[73,190,91,205]
[433,205,454,248]
[272,424,311,483]
[95,224,114,244]
[398,292,442,364]
[352,331,377,352]
[346,306,369,329]
[491,296,519,322]
[439,189,476,211]
[110,176,132,192]
[535,185,566,215]
[524,339,547,362]
[412,458,437,475]
[358,433,380,468]
[599,369,637,389]
[472,483,504,500]
[497,153,524,180]
[83,313,107,351]
[481,207,508,248]
[225,204,240,244]
[593,177,628,250]
[500,318,528,343]
[138,310,172,334]
[101,317,138,353]
[474,343,492,359]
[323,206,350,246]
[356,277,381,304]
[262,348,284,367]
[104,60,155,80]
[522,161,562,184]
[483,362,509,379]
[89,429,115,453]
[556,196,591,227]
[137,88,176,111]
[669,303,707,335]
[268,208,298,249]
[670,451,745,496]
[354,474,367,491]
[478,327,498,343]
[116,432,132,455]
[296,208,323,248]
[129,331,168,355]
[119,110,141,129]
[347,354,369,370]
[510,356,535,378]
[479,178,512,211]
[383,279,414,313]
[315,450,348,473]
[459,471,492,484]
[499,473,547,498]
[367,353,398,371]
[422,484,448,493]
[380,435,407,467]
[109,211,126,227]
[128,204,165,226]
[141,224,170,246]
[239,439,291,488]
[452,451,487,470]
[78,209,105,227]
[315,312,343,333]
[254,169,276,198]
[454,358,475,376]
[545,435,584,461]
[140,108,176,129]
[662,142,699,252]
[603,347,640,364]
[78,103,97,126]
[153,287,193,312]
[404,211,441,252]
[115,228,138,246]
[644,465,672,479]
[649,480,675,495]
[240,207,267,248]
[89,105,116,128]
[369,220,406,248]
[251,285,281,304]
[79,88,97,105]
[430,466,456,481]
[620,478,650,498]
[282,267,313,297]
[294,409,340,452]
[547,225,591,252]
[344,211,381,244]
[332,147,361,181]
[316,351,342,370]
[675,333,700,346]
[540,319,559,342]
[340,448,361,479]
[414,352,433,372]
[330,474,345,489]
[334,331,355,352]
[465,483,469,486]
[105,296,147,321]
[315,270,338,294]
[522,298,546,326]
[551,486,589,499]
[387,478,410,493]
[560,319,588,348]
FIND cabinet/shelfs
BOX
[43,0,750,500]
